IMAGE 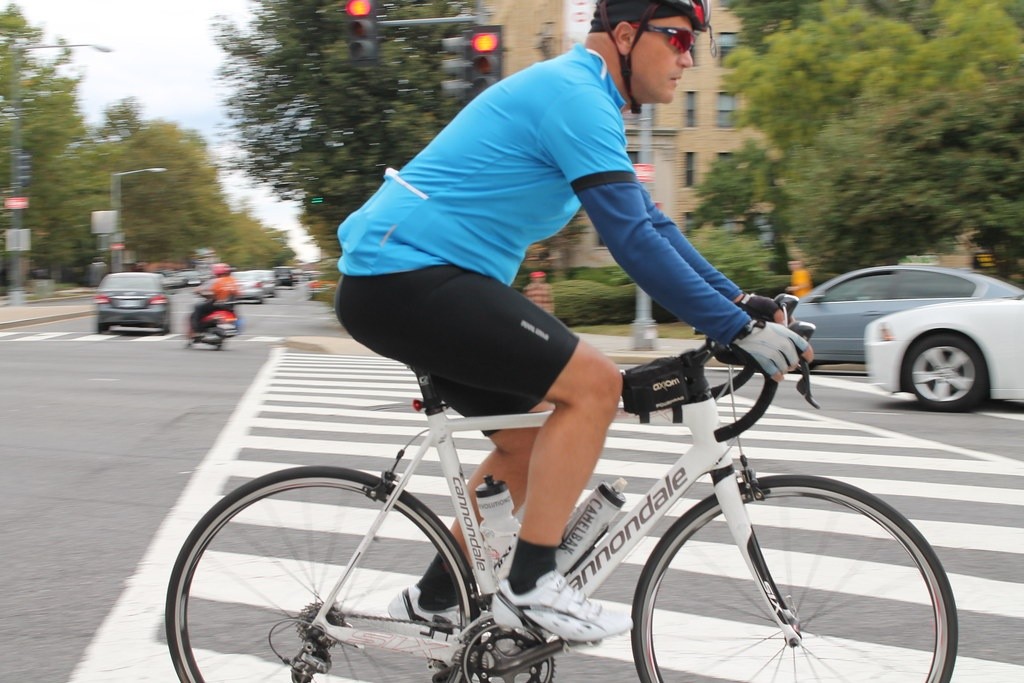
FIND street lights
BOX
[7,41,115,304]
[110,165,170,274]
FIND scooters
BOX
[187,286,239,352]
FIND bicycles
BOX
[163,291,961,683]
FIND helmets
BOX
[665,0,712,31]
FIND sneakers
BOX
[387,585,490,645]
[491,572,637,645]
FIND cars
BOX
[860,292,1023,413]
[156,263,322,305]
[704,263,1024,375]
[93,268,174,335]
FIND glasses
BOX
[636,21,695,55]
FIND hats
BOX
[213,262,231,278]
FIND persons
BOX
[787,257,812,296]
[337,1,813,640]
[524,272,554,315]
[184,262,238,348]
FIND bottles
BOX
[555,477,628,576]
[473,474,521,583]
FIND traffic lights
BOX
[467,23,504,96]
[434,34,472,101]
[346,0,380,62]
